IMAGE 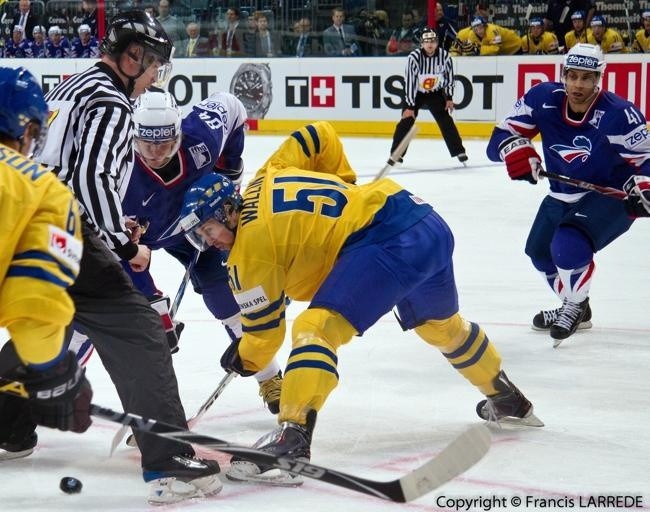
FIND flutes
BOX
[89,403,492,504]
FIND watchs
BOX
[229,61,272,120]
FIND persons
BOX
[0,66,92,434]
[1,6,219,481]
[178,119,533,486]
[390,24,468,166]
[57,88,286,415]
[486,42,649,340]
[0,0,650,58]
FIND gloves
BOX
[498,135,544,185]
[624,174,650,218]
[220,337,256,376]
[23,351,92,433]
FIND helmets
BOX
[179,172,242,253]
[562,43,607,77]
[13,25,24,39]
[0,66,48,139]
[78,24,91,33]
[420,26,439,43]
[571,11,585,20]
[530,17,544,28]
[33,26,45,36]
[591,16,606,27]
[48,26,61,35]
[101,11,173,89]
[132,90,182,169]
[471,16,485,27]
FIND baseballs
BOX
[62,476,84,494]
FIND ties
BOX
[21,14,24,25]
[227,26,234,48]
[339,28,344,44]
[189,41,194,54]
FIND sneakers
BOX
[476,390,532,421]
[458,152,468,162]
[230,427,310,473]
[258,370,283,414]
[1,430,37,451]
[531,295,592,341]
[143,453,219,481]
